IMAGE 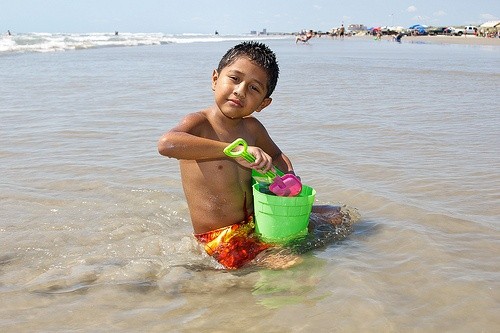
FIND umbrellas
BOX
[409,24,425,29]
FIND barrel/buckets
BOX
[253,183,316,244]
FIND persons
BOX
[374,30,382,39]
[313,32,317,37]
[475,28,500,38]
[331,24,345,36]
[387,29,389,34]
[397,32,404,42]
[318,31,321,37]
[296,29,313,42]
[215,32,218,34]
[348,30,353,36]
[158,41,340,270]
[463,27,467,38]
[115,31,118,35]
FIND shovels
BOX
[223,138,300,197]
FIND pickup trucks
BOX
[448,26,478,36]
